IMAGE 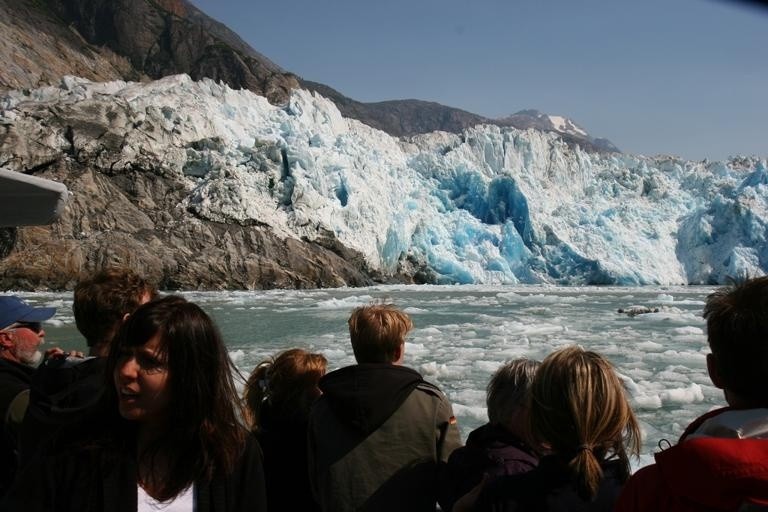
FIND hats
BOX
[1,296,56,330]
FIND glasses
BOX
[8,321,41,334]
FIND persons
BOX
[242,304,464,511]
[1,268,267,510]
[453,344,641,511]
[615,269,767,511]
